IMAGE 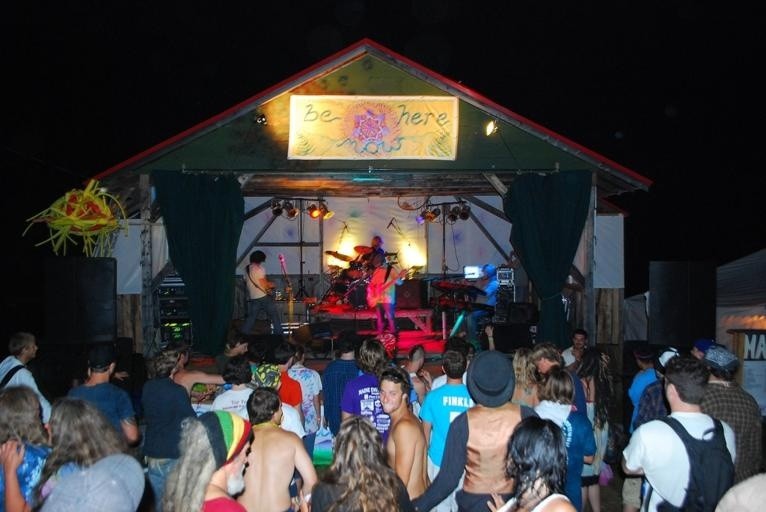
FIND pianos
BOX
[429,296,495,315]
[430,281,487,296]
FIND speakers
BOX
[70,257,116,338]
[649,260,716,345]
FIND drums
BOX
[345,268,363,279]
[349,278,370,309]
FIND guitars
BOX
[366,274,401,308]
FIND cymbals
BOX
[325,251,352,261]
[327,265,343,270]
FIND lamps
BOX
[270,197,336,220]
[416,201,471,227]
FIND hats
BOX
[654,347,680,375]
[87,343,121,369]
[466,350,515,408]
[37,454,145,512]
[693,337,712,355]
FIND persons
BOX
[241,251,283,335]
[354,236,406,335]
[466,264,501,337]
[2,327,765,511]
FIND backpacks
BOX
[655,416,735,512]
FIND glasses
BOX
[381,371,407,385]
